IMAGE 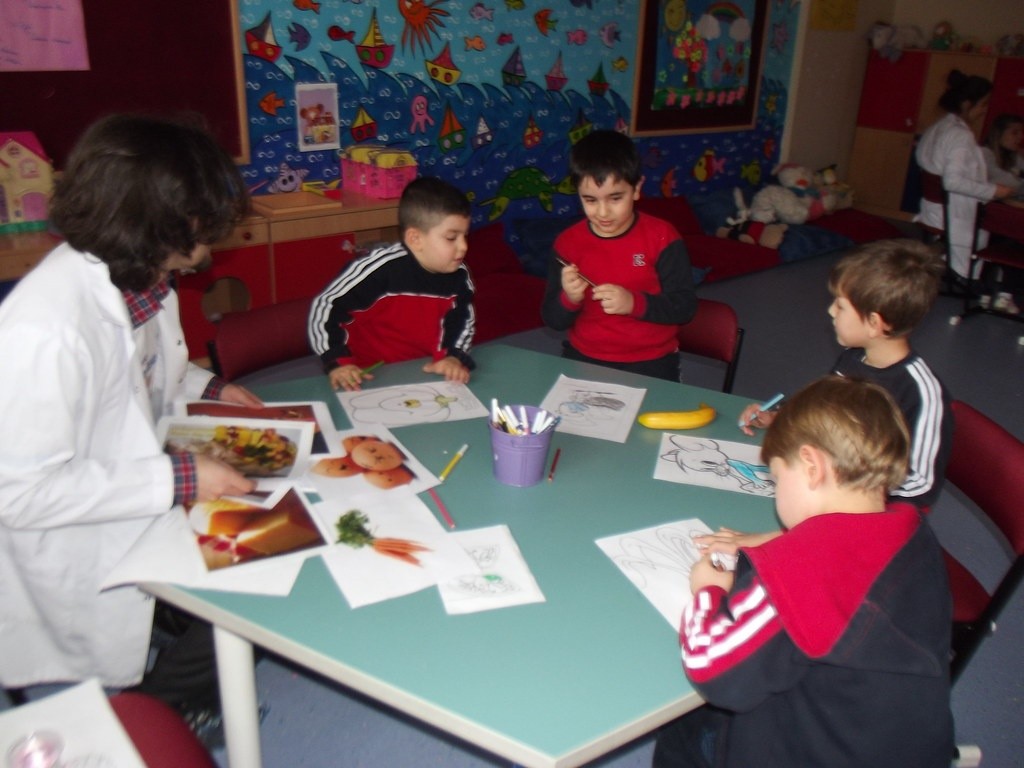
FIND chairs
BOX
[1,681,217,768]
[917,167,1024,326]
[925,399,1024,688]
[680,298,745,395]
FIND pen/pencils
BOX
[429,489,455,528]
[492,398,561,437]
[739,395,783,426]
[548,448,561,481]
[713,554,724,571]
[555,258,596,288]
[352,361,384,381]
[439,444,468,481]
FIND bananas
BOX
[639,402,716,430]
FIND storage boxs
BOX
[339,145,418,199]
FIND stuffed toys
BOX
[686,163,852,250]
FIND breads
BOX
[310,436,414,489]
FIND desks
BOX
[130,343,783,767]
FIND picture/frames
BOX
[630,0,771,138]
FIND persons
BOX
[305,178,476,389]
[651,376,953,768]
[912,71,1020,316]
[0,116,268,744]
[738,237,953,513]
[542,129,697,382]
[982,112,1024,198]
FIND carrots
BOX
[372,537,434,568]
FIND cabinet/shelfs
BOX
[1,191,404,385]
[846,47,1024,220]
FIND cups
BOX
[488,405,555,487]
